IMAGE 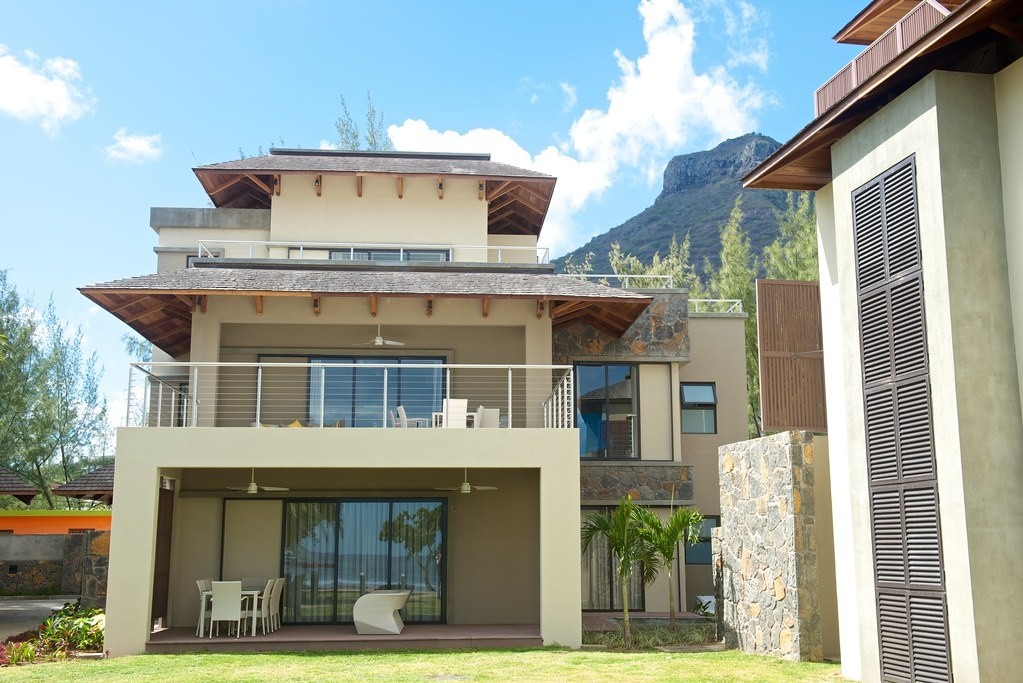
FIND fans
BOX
[225,468,291,494]
[432,468,499,494]
[351,325,406,347]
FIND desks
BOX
[199,586,264,637]
[432,411,476,428]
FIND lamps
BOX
[479,184,484,190]
[315,179,319,186]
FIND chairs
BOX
[390,405,431,427]
[196,578,286,638]
[442,398,468,427]
[470,405,501,428]
